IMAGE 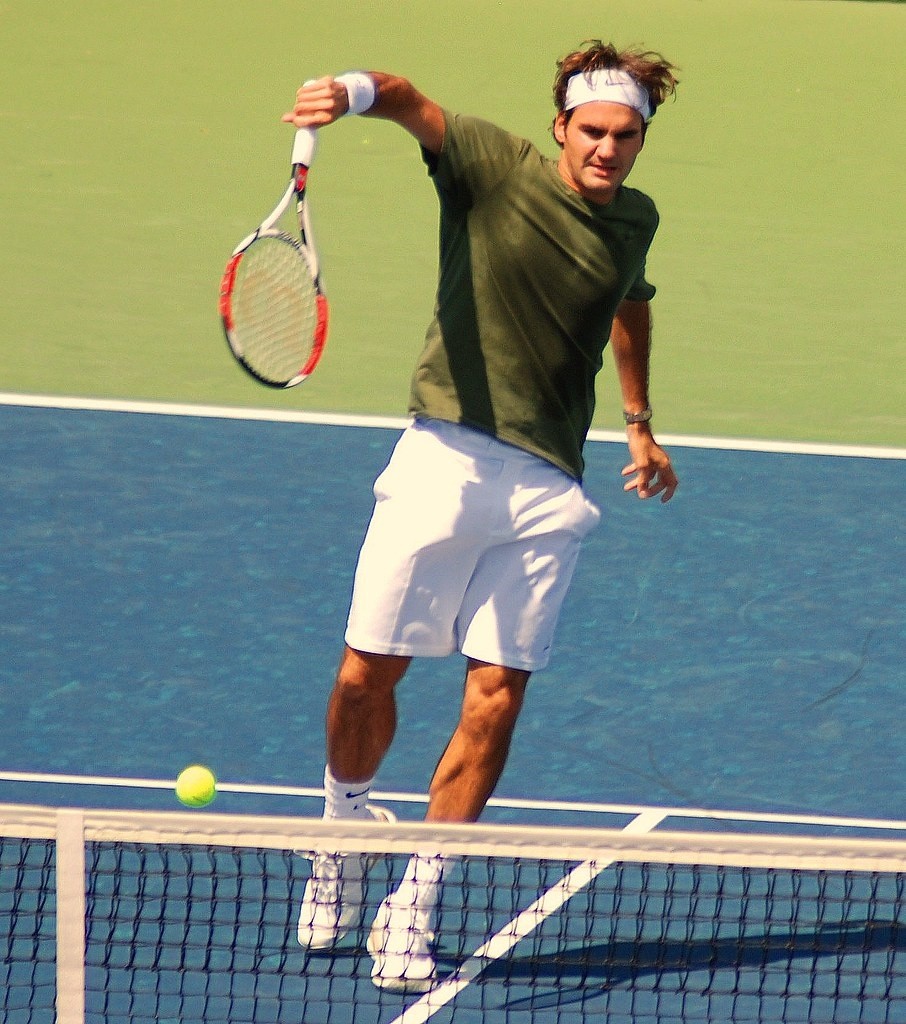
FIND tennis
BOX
[176,765,215,807]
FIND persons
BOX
[281,40,680,994]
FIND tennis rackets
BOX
[220,79,325,390]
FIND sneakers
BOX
[293,806,398,950]
[367,892,437,993]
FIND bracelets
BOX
[623,407,651,423]
[336,72,376,115]
